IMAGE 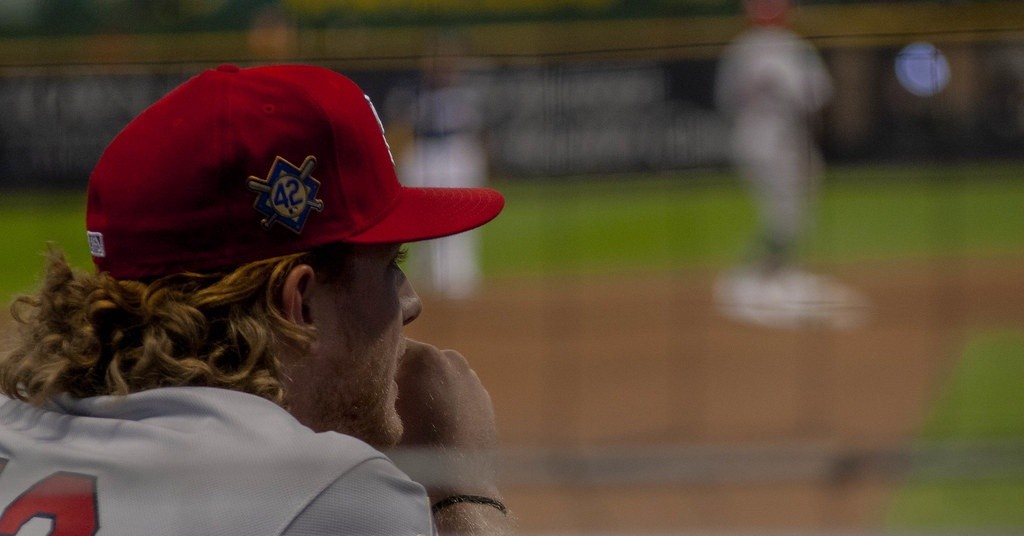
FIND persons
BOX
[1,65,508,536]
[405,42,490,312]
[715,0,838,292]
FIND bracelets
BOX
[430,495,507,513]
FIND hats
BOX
[85,62,508,280]
